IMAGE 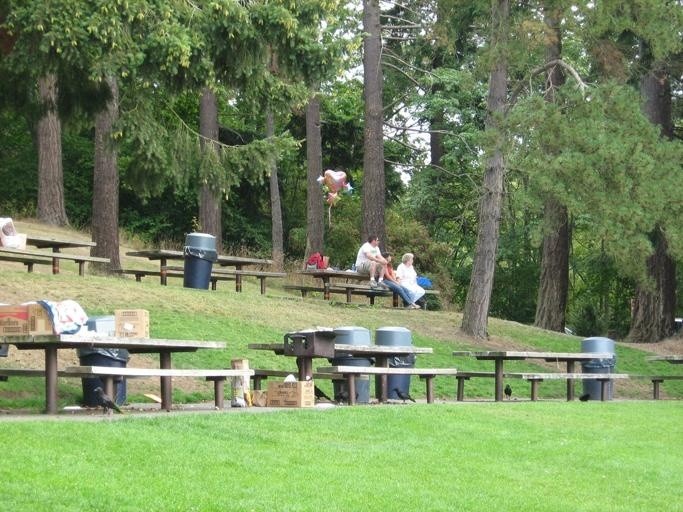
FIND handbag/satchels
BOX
[417,277,433,289]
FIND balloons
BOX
[316,170,352,206]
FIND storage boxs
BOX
[0,305,150,338]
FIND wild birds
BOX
[504,384,512,400]
[93,387,124,414]
[314,386,335,403]
[392,388,416,403]
[579,393,590,402]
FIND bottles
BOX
[352,263,355,272]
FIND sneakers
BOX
[378,281,388,289]
[369,280,378,288]
[411,303,420,309]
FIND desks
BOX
[643,354,683,364]
[0,235,96,274]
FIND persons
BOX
[394,253,425,310]
[381,252,421,309]
[354,233,389,291]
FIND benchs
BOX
[629,375,683,400]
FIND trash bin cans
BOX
[375,327,414,398]
[184,232,217,289]
[77,348,131,405]
[581,337,616,400]
[332,326,372,403]
